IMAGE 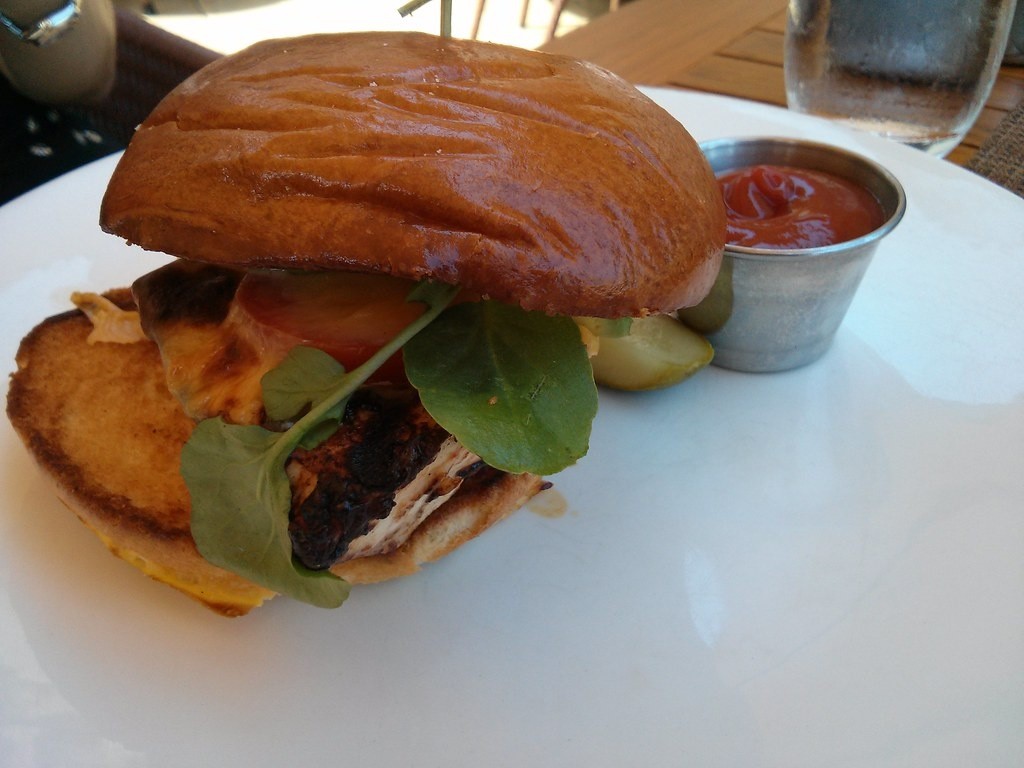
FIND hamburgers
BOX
[5,26,728,613]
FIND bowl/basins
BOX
[697,136,906,374]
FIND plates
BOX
[0,85,1024,768]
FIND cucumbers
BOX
[573,312,716,393]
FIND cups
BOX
[784,0,1016,158]
